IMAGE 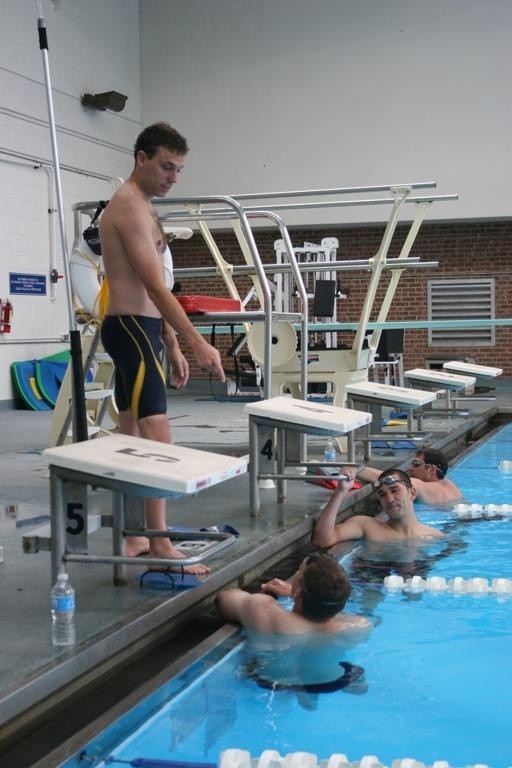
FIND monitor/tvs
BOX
[374,329,405,362]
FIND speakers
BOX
[312,280,336,317]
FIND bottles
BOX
[323,441,336,474]
[49,573,76,647]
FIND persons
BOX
[215,551,375,711]
[356,446,462,504]
[99,122,226,573]
[311,465,467,614]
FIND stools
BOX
[40,432,247,597]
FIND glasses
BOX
[300,551,338,565]
[412,459,444,473]
[374,476,412,487]
